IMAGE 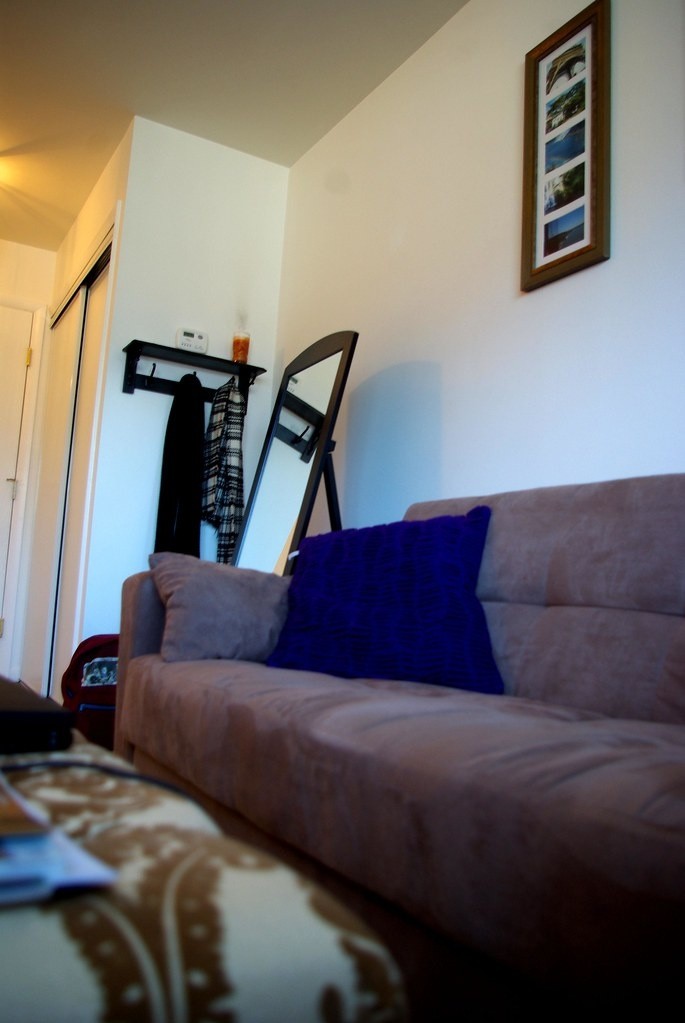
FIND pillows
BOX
[145,551,291,662]
[266,508,505,696]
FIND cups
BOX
[232,332,251,365]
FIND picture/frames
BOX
[519,0,611,294]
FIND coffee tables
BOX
[0,724,400,1023]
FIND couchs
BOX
[118,474,684,1000]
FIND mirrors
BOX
[236,329,360,572]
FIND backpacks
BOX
[61,634,119,732]
